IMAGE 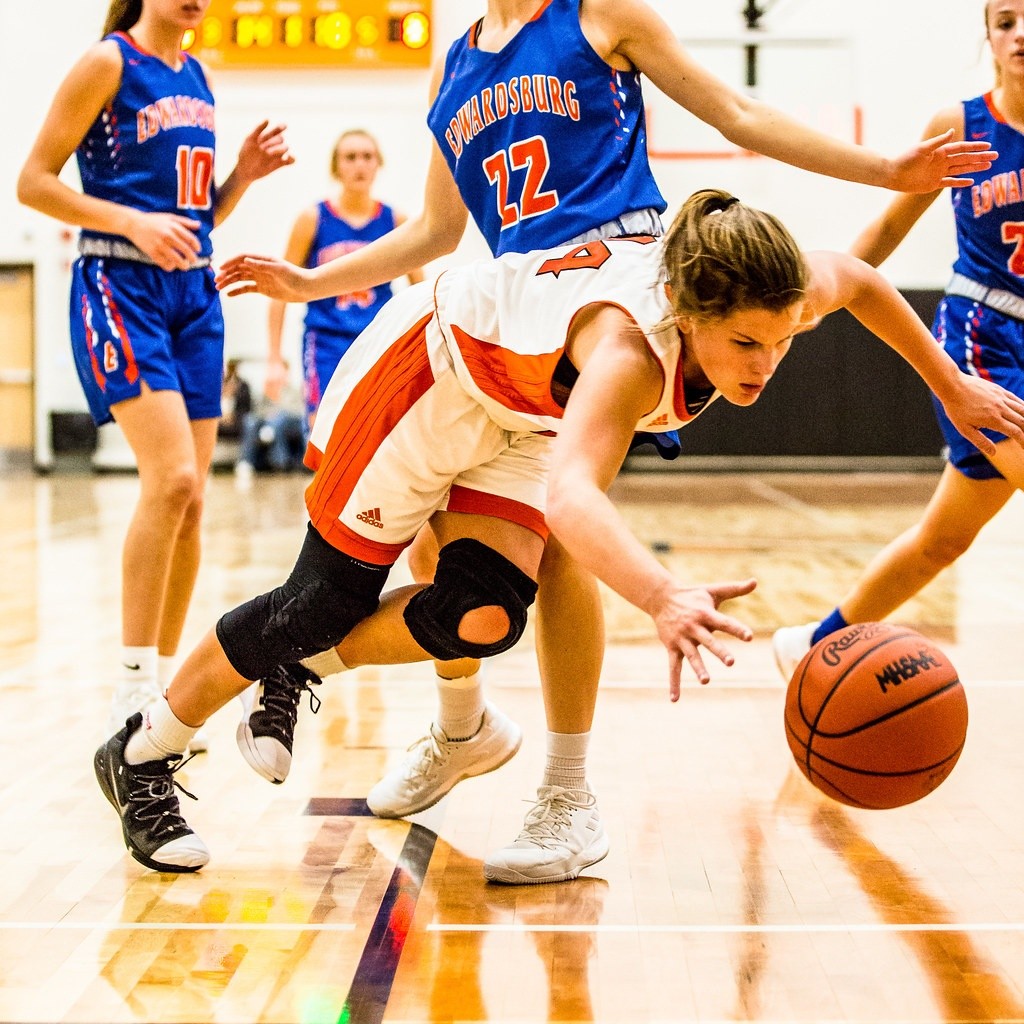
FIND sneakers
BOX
[480,789,610,889]
[236,662,320,788]
[111,678,208,753]
[370,820,488,919]
[487,878,606,946]
[364,702,529,813]
[95,711,211,874]
[776,617,821,674]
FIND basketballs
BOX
[785,621,970,810]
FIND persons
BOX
[217,355,291,488]
[266,130,424,446]
[773,0,1023,665]
[94,188,1024,874]
[213,1,997,884]
[16,0,295,755]
